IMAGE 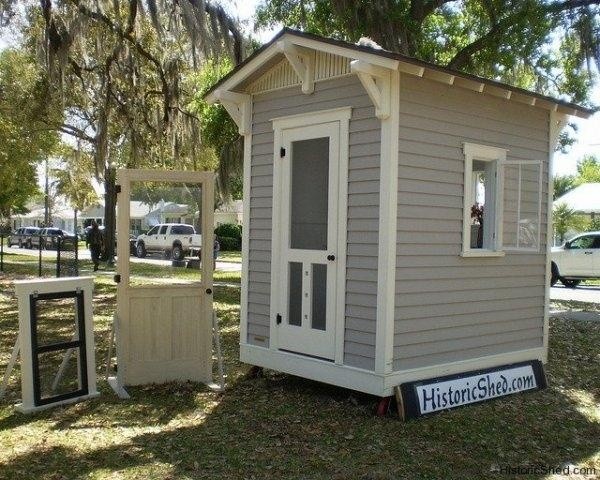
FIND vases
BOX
[476,223,485,248]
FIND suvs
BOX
[551,231,600,289]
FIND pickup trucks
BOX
[134,223,202,261]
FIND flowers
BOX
[468,204,487,226]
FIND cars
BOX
[8,225,106,249]
[87,230,137,255]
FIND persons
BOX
[86,222,104,271]
